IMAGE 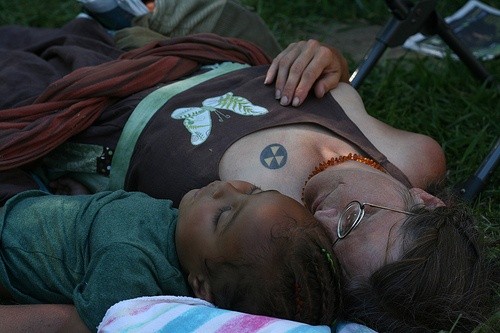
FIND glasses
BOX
[330,200,418,249]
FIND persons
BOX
[0,20,495,333]
[0,180,349,333]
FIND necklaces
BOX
[300,151,388,209]
